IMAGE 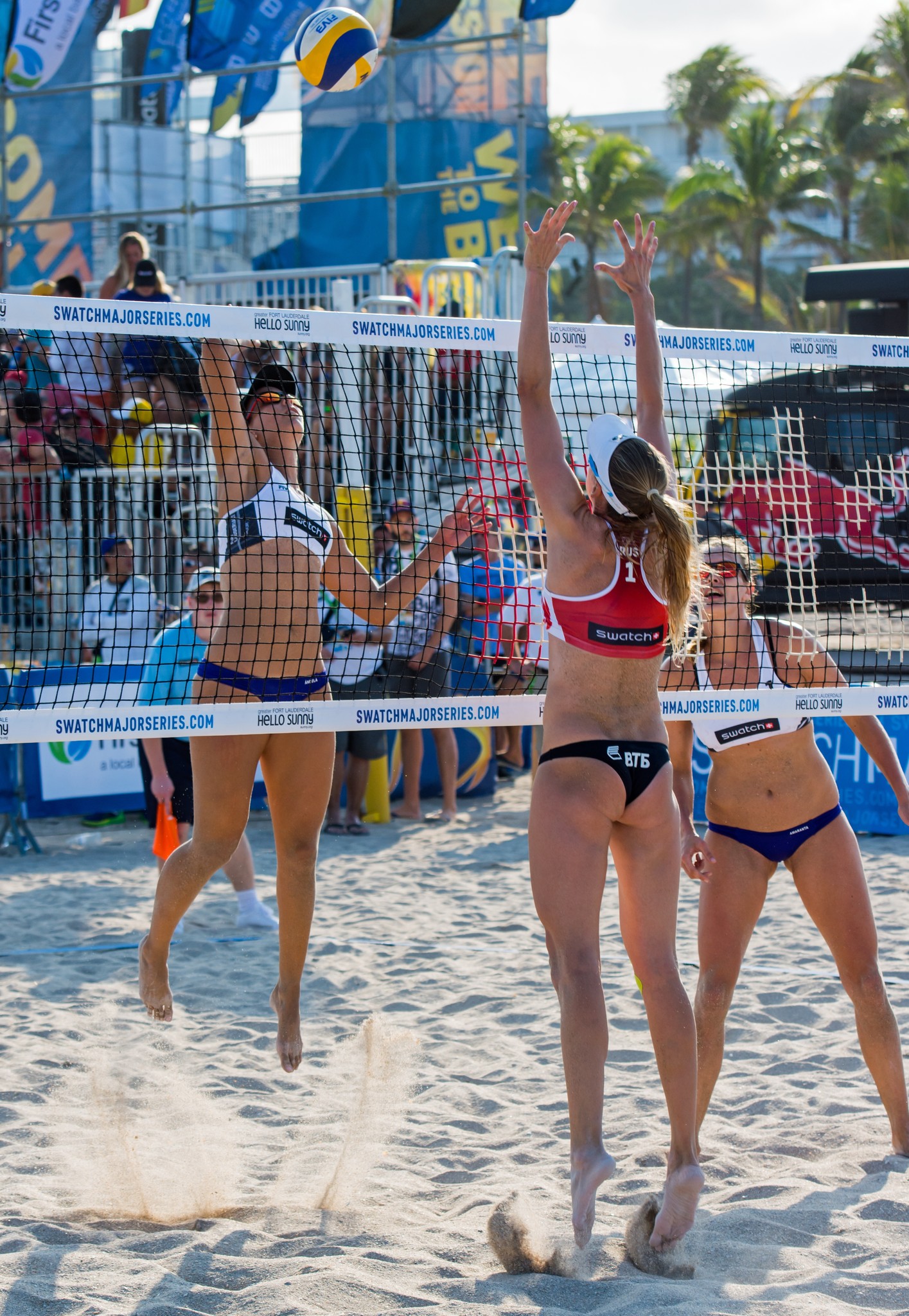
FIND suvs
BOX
[685,366,909,618]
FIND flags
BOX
[1,0,580,137]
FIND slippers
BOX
[425,814,451,823]
[494,752,523,772]
[323,822,347,835]
[347,822,370,835]
[391,809,400,818]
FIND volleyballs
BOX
[293,6,379,93]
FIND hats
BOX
[100,533,126,556]
[385,497,416,520]
[132,258,158,288]
[53,404,81,422]
[586,413,647,519]
[240,363,296,414]
[186,567,221,591]
[110,398,152,424]
[528,529,547,541]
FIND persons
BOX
[0,231,562,838]
[138,337,492,1072]
[658,535,909,1166]
[518,199,705,1252]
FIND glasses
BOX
[692,560,752,583]
[195,593,223,604]
[246,392,303,421]
[474,531,489,538]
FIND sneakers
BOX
[173,915,183,935]
[236,902,279,931]
